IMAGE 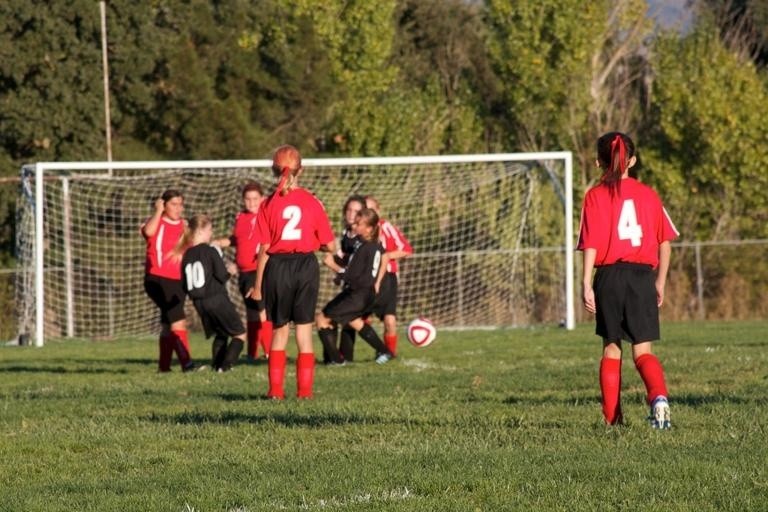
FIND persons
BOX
[242,141,336,400]
[574,130,682,432]
[140,182,268,373]
[316,194,416,367]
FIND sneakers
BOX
[649,396,671,431]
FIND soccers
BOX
[407,317,436,348]
